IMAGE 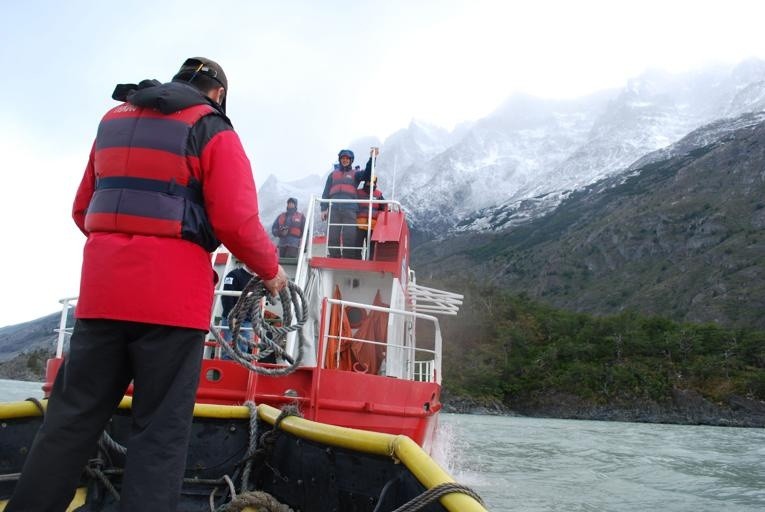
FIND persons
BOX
[356,174,388,259]
[271,197,308,258]
[221,262,258,360]
[320,147,379,259]
[2,57,288,512]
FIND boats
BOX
[42,148,463,447]
[0,396,490,512]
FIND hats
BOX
[287,198,297,208]
[178,56,227,114]
[339,151,354,165]
